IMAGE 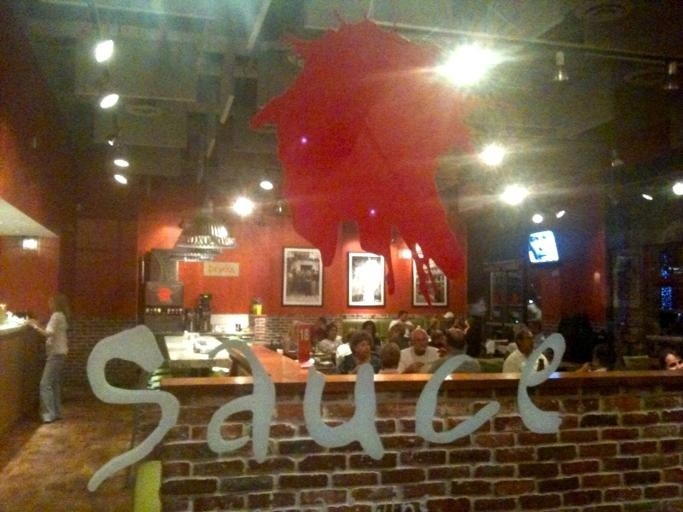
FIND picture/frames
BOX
[346,250,386,308]
[281,245,324,308]
[411,253,449,308]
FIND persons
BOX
[24,292,72,423]
[310,287,549,375]
[0,304,24,329]
[659,347,683,370]
[576,345,608,373]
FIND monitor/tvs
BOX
[525,227,561,269]
